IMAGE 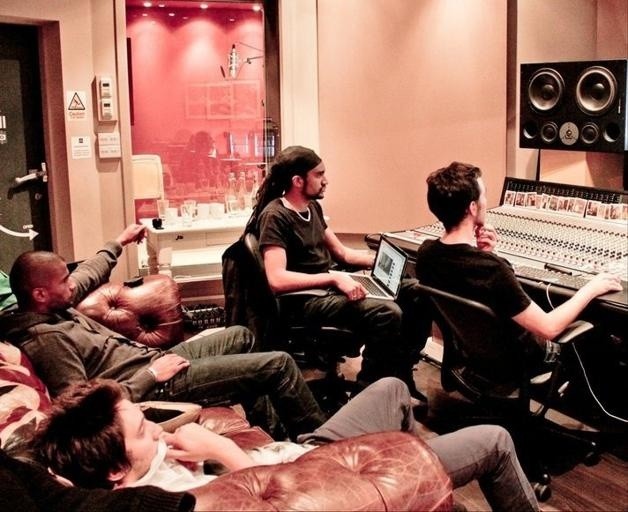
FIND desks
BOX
[363,230,628,437]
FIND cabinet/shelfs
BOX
[137,209,328,284]
[183,79,262,120]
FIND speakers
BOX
[520,59,628,154]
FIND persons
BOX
[413,162,625,390]
[1,222,329,442]
[258,147,434,405]
[179,130,219,180]
[31,373,540,510]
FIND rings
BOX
[352,294,357,298]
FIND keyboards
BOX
[514,264,591,290]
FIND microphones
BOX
[229,44,239,78]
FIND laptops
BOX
[328,235,409,301]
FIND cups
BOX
[193,203,210,220]
[181,200,196,223]
[152,219,162,229]
[164,208,177,230]
[157,200,169,221]
[210,203,225,220]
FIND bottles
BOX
[224,173,239,218]
[251,172,260,210]
[238,172,251,217]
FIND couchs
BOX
[1,261,467,512]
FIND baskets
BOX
[185,303,225,330]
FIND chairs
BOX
[239,232,368,416]
[412,283,599,502]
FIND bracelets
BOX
[147,367,157,383]
